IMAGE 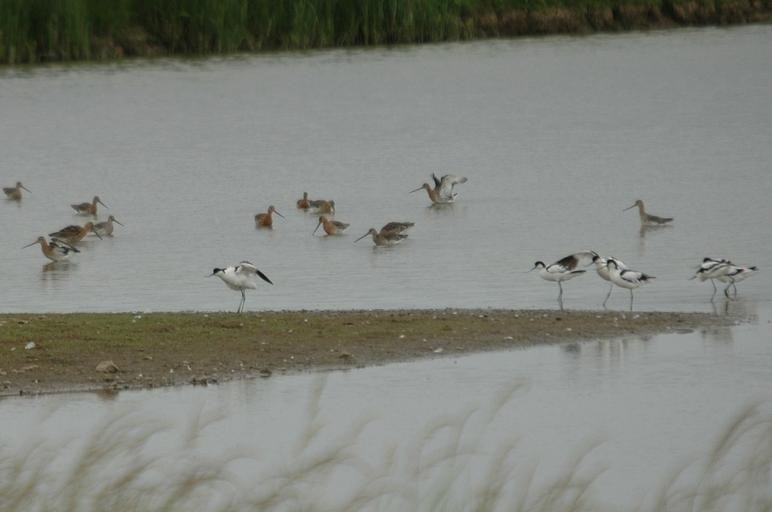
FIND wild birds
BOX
[352,220,415,245]
[1,181,30,201]
[20,235,80,262]
[408,172,468,204]
[526,249,600,294]
[254,204,287,227]
[597,259,655,297]
[312,215,350,235]
[205,259,274,301]
[703,257,759,295]
[311,199,336,212]
[589,250,629,297]
[52,221,104,245]
[297,192,312,209]
[91,215,124,237]
[623,199,675,227]
[688,259,730,295]
[70,195,108,215]
